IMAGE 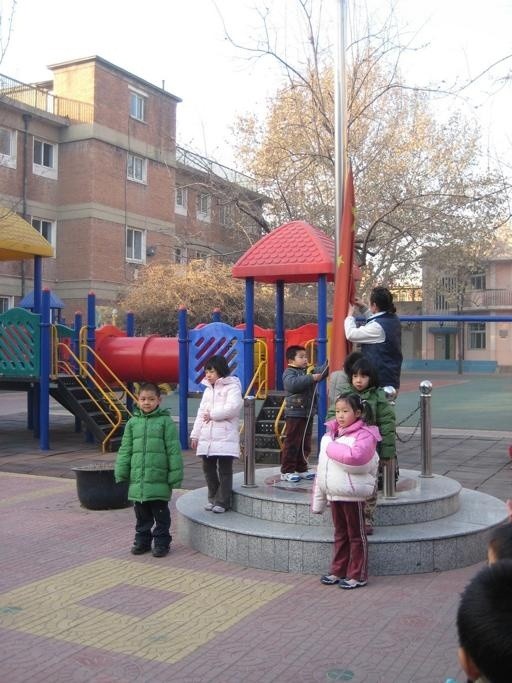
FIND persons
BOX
[310,391,381,588]
[113,381,184,557]
[486,522,511,569]
[454,560,511,681]
[187,355,245,513]
[344,286,403,390]
[279,345,320,483]
[325,350,397,495]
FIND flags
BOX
[327,168,355,371]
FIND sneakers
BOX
[338,577,367,589]
[280,473,301,482]
[365,525,373,534]
[152,545,170,557]
[204,503,214,511]
[321,573,346,585]
[131,542,151,555]
[211,505,226,513]
[295,471,315,480]
